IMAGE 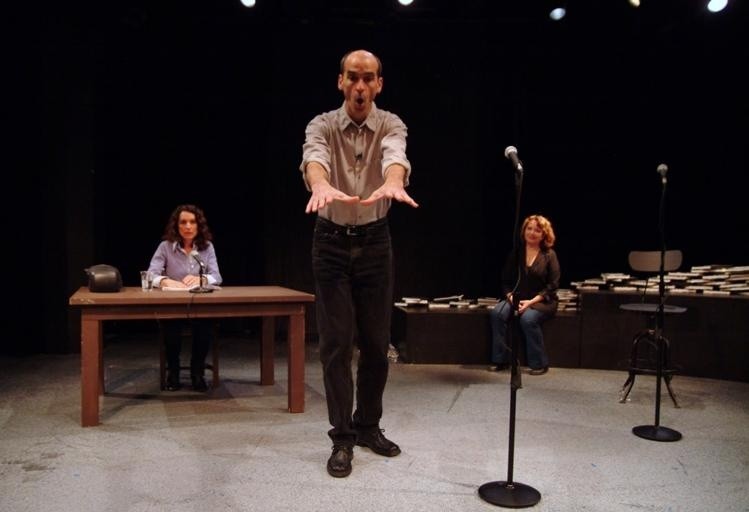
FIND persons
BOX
[145,205,223,392]
[299,49,420,479]
[488,214,561,376]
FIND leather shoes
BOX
[353,425,403,457]
[488,364,509,371]
[530,366,548,375]
[327,440,354,477]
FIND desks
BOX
[68,285,316,428]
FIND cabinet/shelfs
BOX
[395,301,581,369]
[577,288,749,383]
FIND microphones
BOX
[190,249,206,269]
[503,144,524,173]
[656,162,669,184]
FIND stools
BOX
[154,319,221,389]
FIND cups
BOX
[140,271,154,292]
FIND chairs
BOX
[618,249,688,409]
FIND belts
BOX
[317,218,391,237]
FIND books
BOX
[570,265,749,295]
[393,294,501,310]
[556,289,579,311]
